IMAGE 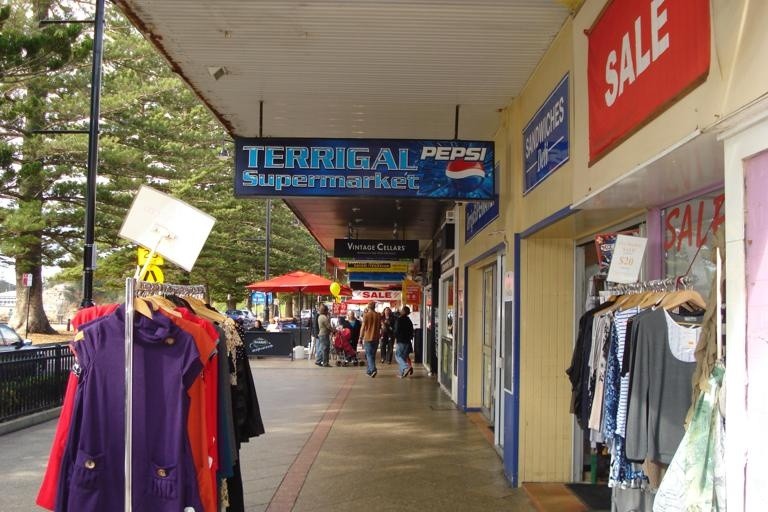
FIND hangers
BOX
[592,275,709,322]
[73,280,228,344]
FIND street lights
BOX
[227,293,231,310]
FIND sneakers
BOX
[367,368,377,378]
[315,361,329,367]
[403,366,413,377]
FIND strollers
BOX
[332,328,365,367]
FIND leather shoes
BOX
[381,358,391,364]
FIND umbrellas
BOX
[245,270,352,346]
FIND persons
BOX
[249,317,282,332]
[309,301,413,378]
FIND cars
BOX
[225,309,256,321]
[301,310,314,318]
[266,317,309,332]
[0,322,47,375]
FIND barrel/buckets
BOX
[294,345,304,359]
